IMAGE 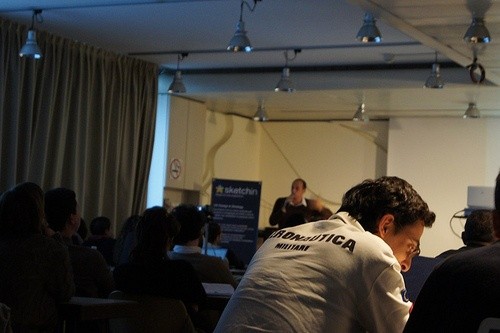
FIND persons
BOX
[0,172,500,333]
[214,176,436,333]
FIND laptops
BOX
[199,247,229,260]
[467,186,496,210]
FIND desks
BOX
[53,296,143,333]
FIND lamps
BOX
[167,53,190,93]
[462,19,491,44]
[353,13,383,44]
[273,50,297,93]
[252,106,268,121]
[463,104,480,119]
[351,104,370,123]
[226,0,263,52]
[423,53,444,89]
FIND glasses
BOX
[393,217,420,258]
